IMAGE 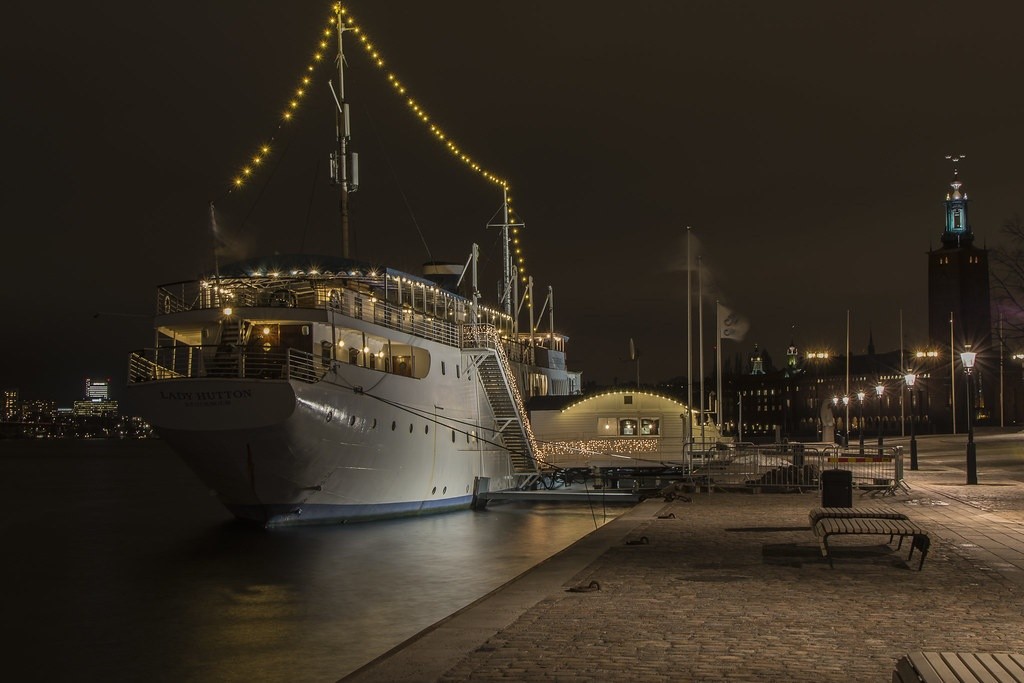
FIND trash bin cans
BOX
[822,469,853,508]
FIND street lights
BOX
[807,351,829,416]
[875,380,885,458]
[903,366,919,470]
[960,341,978,485]
[857,389,866,457]
[917,344,938,434]
[843,395,850,450]
[832,395,839,444]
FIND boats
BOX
[109,1,584,531]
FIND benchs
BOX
[808,507,910,551]
[814,518,931,571]
[892,652,1024,683]
